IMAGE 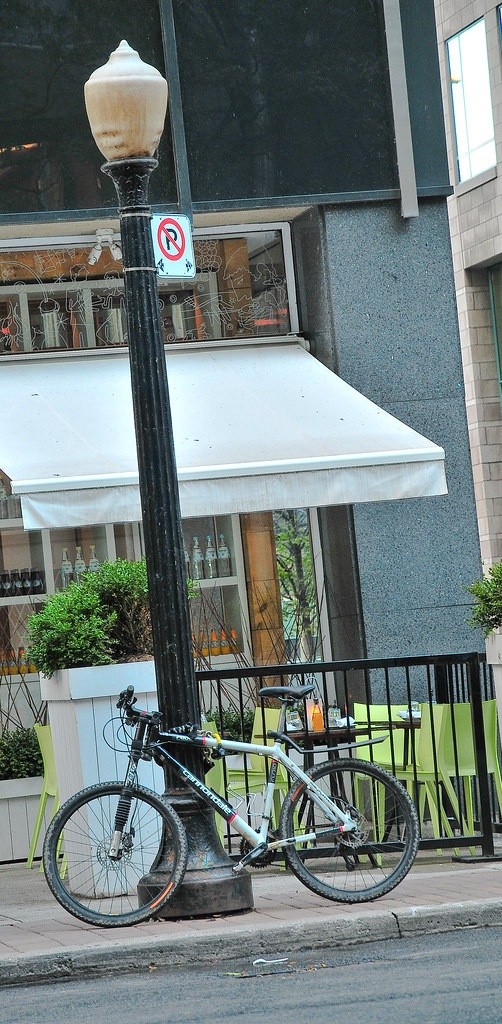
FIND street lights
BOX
[83,36,266,922]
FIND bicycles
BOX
[43,681,420,928]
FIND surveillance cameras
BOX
[109,238,123,261]
[87,244,103,266]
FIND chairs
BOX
[24,699,500,869]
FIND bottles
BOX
[312,697,324,731]
[210,629,221,655]
[0,567,45,597]
[10,488,21,518]
[191,537,205,579]
[184,547,190,578]
[218,533,230,576]
[0,479,8,518]
[192,633,198,657]
[0,646,38,675]
[230,626,239,654]
[205,536,218,578]
[59,548,72,587]
[220,629,232,654]
[89,545,99,572]
[73,546,86,579]
[198,629,209,657]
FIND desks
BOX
[352,719,452,840]
[251,726,397,844]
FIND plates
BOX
[402,717,422,722]
